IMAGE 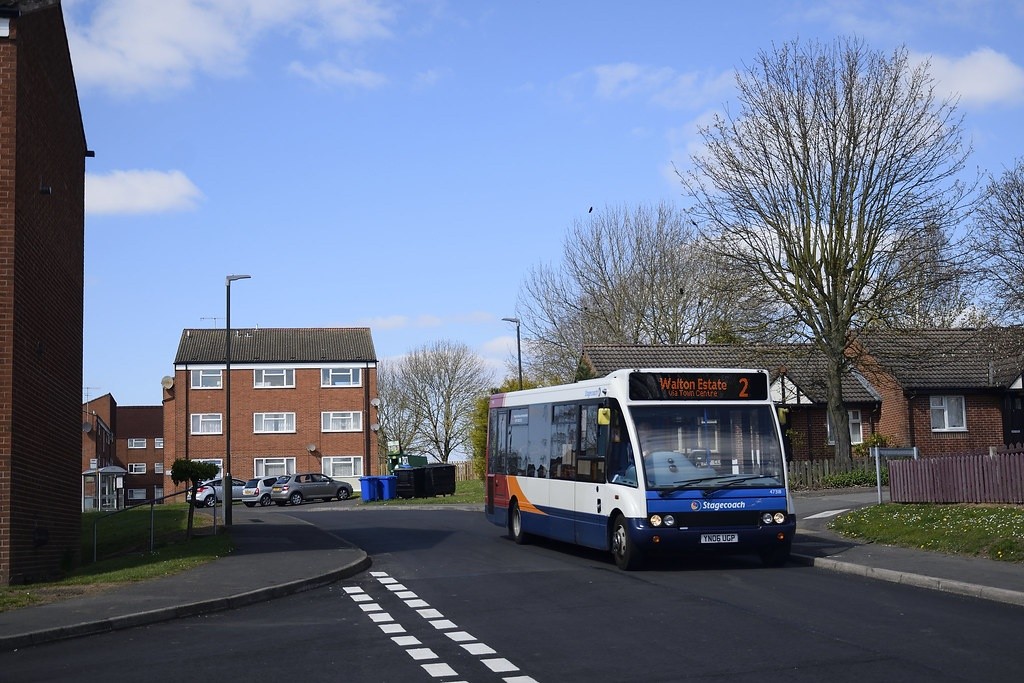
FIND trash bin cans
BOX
[413,463,456,499]
[358,476,378,502]
[394,469,416,499]
[377,476,397,501]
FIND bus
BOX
[483,366,797,570]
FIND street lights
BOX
[503,316,524,390]
[224,274,252,526]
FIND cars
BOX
[186,476,246,508]
[270,472,353,507]
[241,475,287,508]
